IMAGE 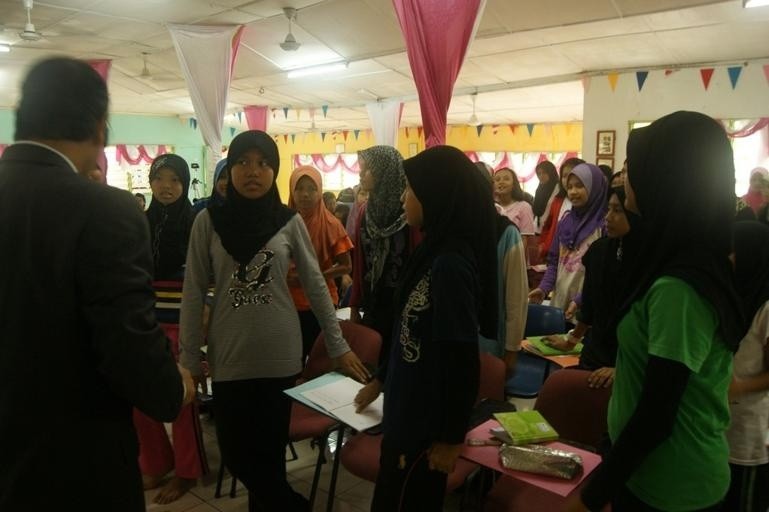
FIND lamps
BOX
[284,59,349,79]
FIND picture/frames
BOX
[407,141,419,157]
[595,157,617,173]
[594,128,617,156]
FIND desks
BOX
[456,420,600,495]
[515,332,584,367]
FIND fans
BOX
[0,1,98,46]
[305,117,319,132]
[461,88,488,129]
[274,6,313,51]
[124,52,173,83]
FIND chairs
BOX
[482,363,615,511]
[501,304,570,405]
[327,353,508,509]
[206,312,383,509]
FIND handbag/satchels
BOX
[499,440,583,482]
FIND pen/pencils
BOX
[468,438,503,448]
